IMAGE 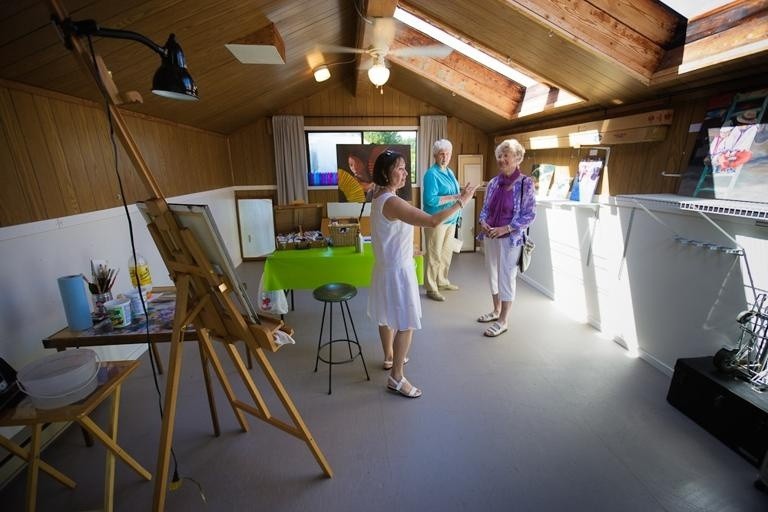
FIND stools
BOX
[312,283,370,396]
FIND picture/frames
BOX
[457,155,483,189]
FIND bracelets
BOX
[456,198,464,208]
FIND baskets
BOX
[305,231,327,249]
[276,235,308,251]
[328,217,361,246]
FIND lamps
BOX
[311,65,331,82]
[366,64,390,90]
[52,18,198,102]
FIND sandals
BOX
[478,313,500,323]
[383,357,410,370]
[387,375,422,398]
[484,323,507,337]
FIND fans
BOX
[304,18,461,68]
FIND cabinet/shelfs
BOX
[237,199,278,263]
[457,197,477,253]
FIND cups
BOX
[87,292,112,320]
[56,274,95,333]
[124,289,151,317]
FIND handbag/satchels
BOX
[518,237,535,273]
[453,226,464,254]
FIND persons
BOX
[708,121,734,173]
[722,110,757,172]
[366,150,479,397]
[423,140,460,300]
[478,137,538,336]
[577,164,587,182]
[347,152,376,192]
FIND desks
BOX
[0,358,151,512]
[260,248,425,315]
[40,280,223,449]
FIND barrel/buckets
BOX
[16,349,104,410]
[125,287,149,314]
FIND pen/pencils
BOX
[80,260,120,302]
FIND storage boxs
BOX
[666,355,768,470]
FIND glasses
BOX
[384,147,393,159]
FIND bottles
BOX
[127,248,154,299]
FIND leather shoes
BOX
[427,289,446,301]
[439,283,458,290]
